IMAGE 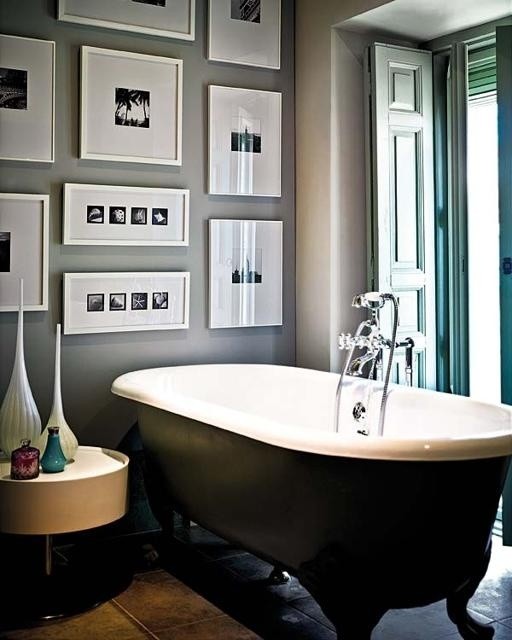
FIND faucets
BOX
[349,344,382,374]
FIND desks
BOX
[0,443,136,629]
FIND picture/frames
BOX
[61,183,190,247]
[60,272,189,336]
[1,34,55,165]
[207,84,283,199]
[0,193,49,312]
[58,0,195,42]
[80,44,182,167]
[206,1,282,70]
[208,217,284,329]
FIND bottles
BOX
[9,440,41,481]
[0,277,47,454]
[37,324,79,463]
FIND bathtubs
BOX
[111,363,512,640]
